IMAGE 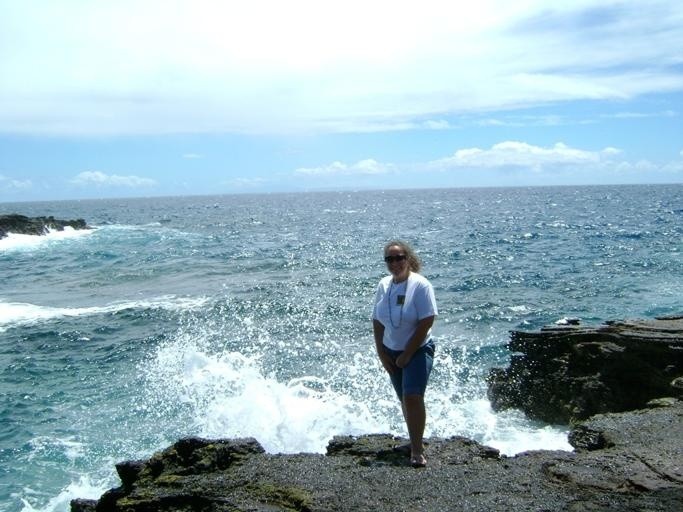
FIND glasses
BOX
[385,255,405,263]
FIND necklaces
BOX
[388,280,407,329]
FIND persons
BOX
[372,241,437,467]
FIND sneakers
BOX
[394,444,423,452]
[410,454,427,467]
